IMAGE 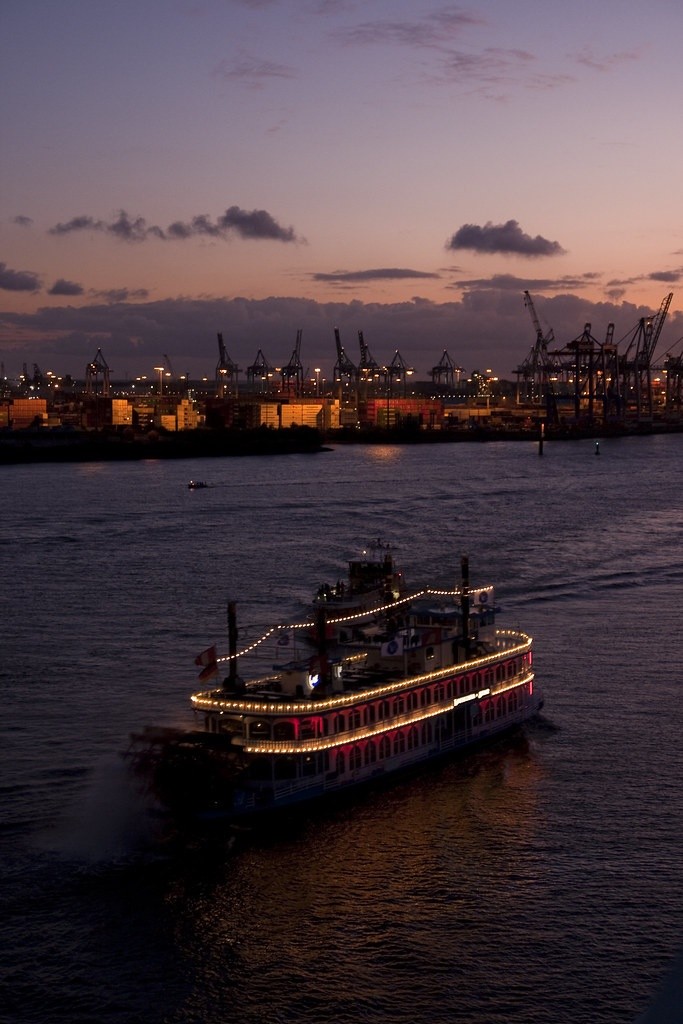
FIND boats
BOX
[302,538,411,645]
[117,552,546,821]
[187,480,209,489]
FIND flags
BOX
[198,660,219,682]
[380,637,403,657]
[421,628,442,645]
[195,645,216,667]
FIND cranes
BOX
[1,290,682,425]
